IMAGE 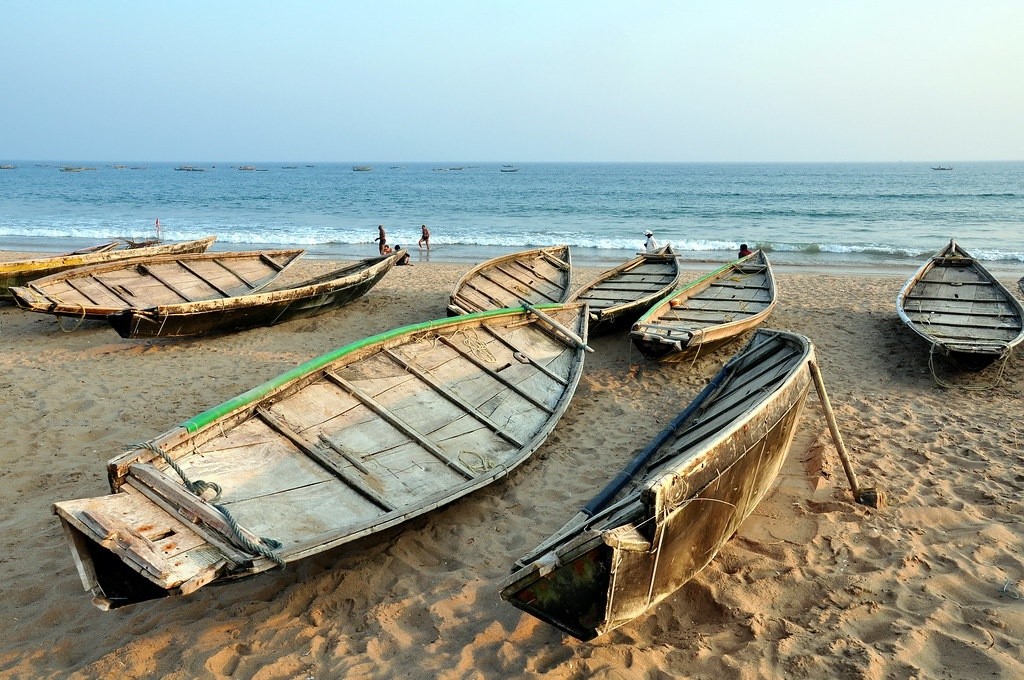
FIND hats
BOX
[644,230,653,235]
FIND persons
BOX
[381,244,391,256]
[737,244,751,260]
[376,225,387,255]
[394,244,410,266]
[642,230,656,253]
[418,224,429,250]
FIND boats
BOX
[629,249,778,364]
[565,244,683,334]
[2,162,521,172]
[500,327,817,643]
[9,249,308,321]
[447,245,573,319]
[106,249,408,339]
[51,303,590,610]
[1,236,218,305]
[932,166,954,170]
[896,238,1024,374]
